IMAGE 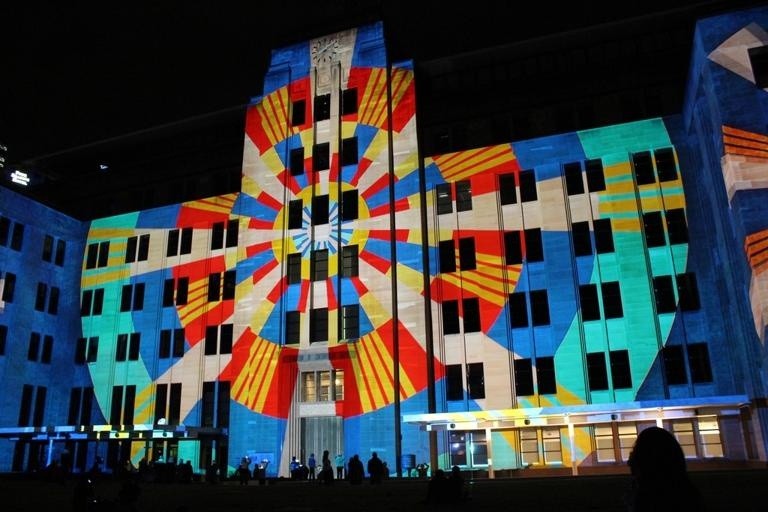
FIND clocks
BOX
[311,38,339,66]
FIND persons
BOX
[625,425,694,512]
[38,450,466,512]
